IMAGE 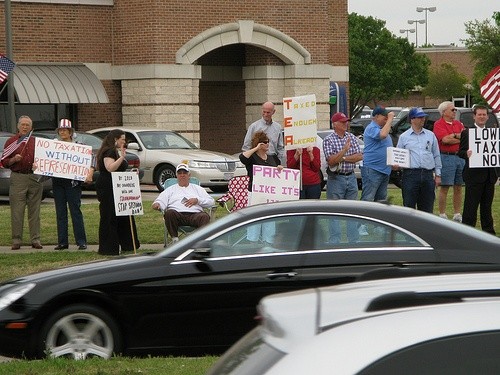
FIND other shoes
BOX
[78,244,87,250]
[357,224,369,236]
[373,226,389,235]
[31,242,43,249]
[11,244,21,250]
[54,244,68,249]
[453,213,462,222]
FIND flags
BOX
[0,130,32,162]
[0,54,16,84]
[481,65,500,113]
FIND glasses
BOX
[450,108,457,112]
[262,140,269,144]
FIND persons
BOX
[396,107,443,217]
[432,102,465,223]
[0,116,43,250]
[152,163,214,244]
[94,128,141,256]
[356,107,397,236]
[321,112,363,244]
[458,104,500,236]
[32,119,97,250]
[239,102,328,247]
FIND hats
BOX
[372,106,391,116]
[175,164,189,173]
[408,105,428,118]
[332,112,352,123]
[56,118,73,134]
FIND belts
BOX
[441,151,458,155]
[13,169,33,174]
[333,171,353,176]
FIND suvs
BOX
[350,106,499,192]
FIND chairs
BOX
[157,178,200,249]
[216,176,263,246]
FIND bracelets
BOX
[342,156,346,161]
[454,133,456,138]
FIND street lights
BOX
[400,29,415,37]
[408,20,425,48]
[416,7,436,45]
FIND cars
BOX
[203,273,500,375]
[232,130,367,190]
[0,130,144,201]
[0,195,500,362]
[86,126,248,192]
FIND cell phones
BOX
[181,197,192,208]
